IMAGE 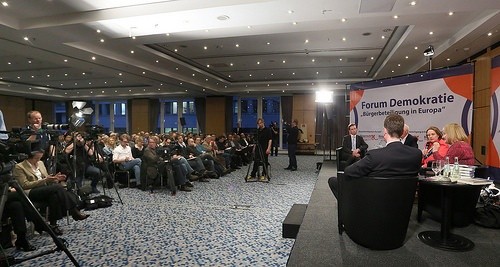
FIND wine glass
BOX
[435,160,445,178]
[432,161,438,178]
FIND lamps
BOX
[314,90,334,160]
[74,110,83,118]
[423,45,435,57]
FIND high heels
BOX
[34,224,59,235]
[15,239,36,251]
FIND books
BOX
[457,178,493,185]
[445,163,477,179]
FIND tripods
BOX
[0,179,81,267]
[83,140,124,205]
[245,143,270,181]
[46,141,73,177]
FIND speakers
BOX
[180,118,186,125]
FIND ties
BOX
[352,136,356,152]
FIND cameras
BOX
[42,122,70,131]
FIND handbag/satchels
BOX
[85,195,113,211]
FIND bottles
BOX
[452,156,460,179]
[443,157,450,178]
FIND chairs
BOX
[418,178,486,252]
[473,166,500,230]
[109,153,167,193]
[336,170,420,251]
[0,191,70,239]
[335,146,367,170]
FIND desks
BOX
[295,143,315,155]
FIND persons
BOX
[270,122,278,156]
[113,135,143,189]
[0,200,36,251]
[14,147,90,235]
[54,129,79,153]
[341,123,368,166]
[143,137,194,193]
[422,127,449,164]
[432,123,475,166]
[123,128,252,182]
[97,131,120,183]
[65,131,104,194]
[327,114,422,200]
[283,118,298,170]
[235,123,242,134]
[21,111,56,176]
[247,117,272,179]
[399,124,419,149]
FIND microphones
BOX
[427,143,439,153]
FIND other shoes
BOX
[210,159,250,178]
[186,182,194,187]
[284,166,290,170]
[181,185,193,191]
[199,177,209,182]
[261,175,265,180]
[248,176,254,179]
[188,175,199,180]
[50,225,63,236]
[73,215,89,222]
[202,170,213,175]
[137,184,146,190]
[290,166,297,172]
